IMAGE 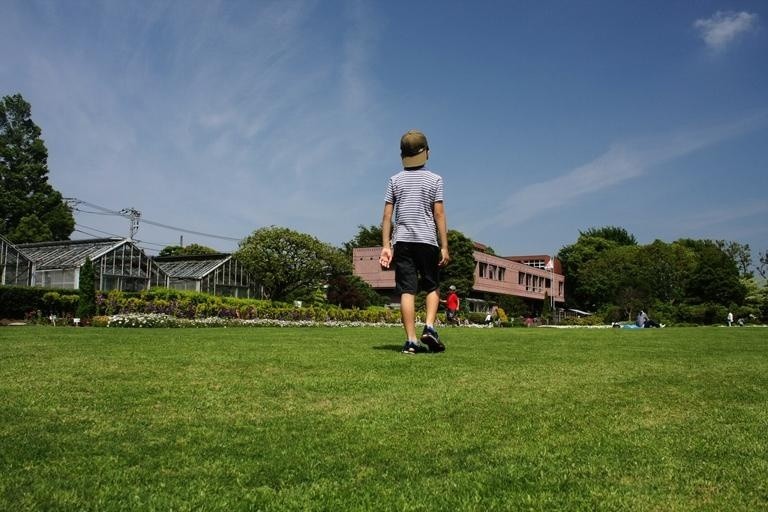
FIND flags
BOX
[544,259,554,270]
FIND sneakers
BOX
[402,342,429,354]
[421,325,445,353]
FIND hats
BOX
[400,131,428,169]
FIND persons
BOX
[439,285,461,328]
[638,311,666,328]
[484,312,491,326]
[725,311,734,326]
[377,129,450,355]
